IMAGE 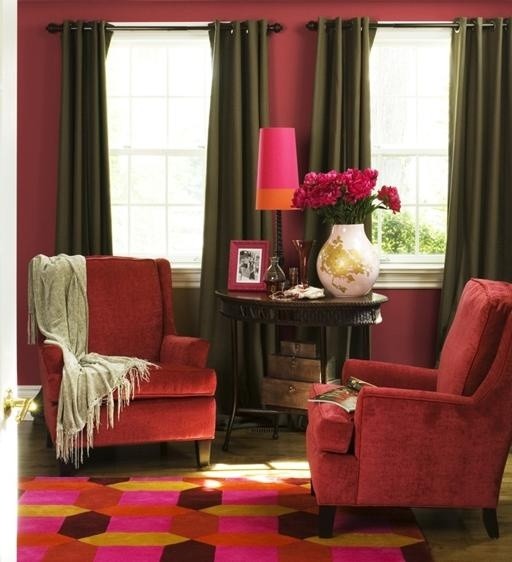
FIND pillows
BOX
[438,278,512,396]
[81,259,164,362]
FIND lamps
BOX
[255,127,303,264]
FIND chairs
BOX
[34,255,219,476]
[307,278,512,537]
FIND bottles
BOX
[263,257,298,295]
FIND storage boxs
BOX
[260,341,339,407]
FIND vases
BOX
[314,222,382,300]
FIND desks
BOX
[215,288,387,452]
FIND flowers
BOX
[293,167,403,224]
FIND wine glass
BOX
[292,239,316,292]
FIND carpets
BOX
[17,475,434,562]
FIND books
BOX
[306,375,379,415]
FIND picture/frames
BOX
[228,241,267,291]
